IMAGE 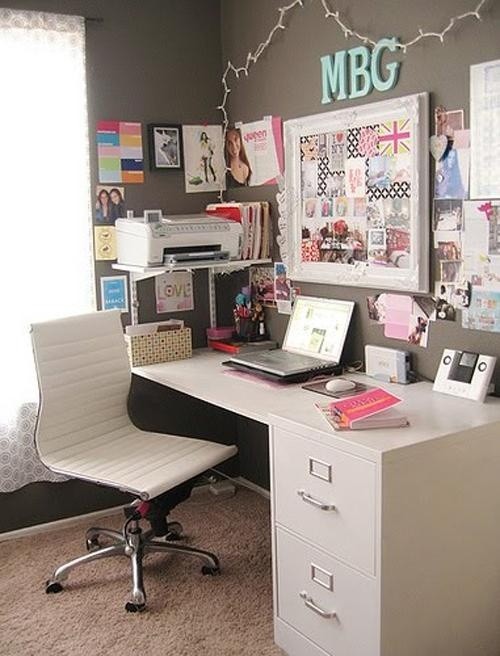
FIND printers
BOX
[114,211,243,266]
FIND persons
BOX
[250,268,273,299]
[453,207,462,230]
[95,189,123,225]
[274,264,290,300]
[437,150,463,197]
[223,127,253,188]
[200,133,216,183]
[438,245,459,260]
[434,208,444,229]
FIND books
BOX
[313,385,410,431]
[207,200,271,259]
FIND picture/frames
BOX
[148,123,184,172]
[283,90,430,295]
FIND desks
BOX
[128,346,500,656]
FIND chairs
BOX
[29,309,239,615]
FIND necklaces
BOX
[231,159,243,168]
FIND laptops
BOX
[220,294,357,383]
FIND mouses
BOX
[326,377,357,392]
